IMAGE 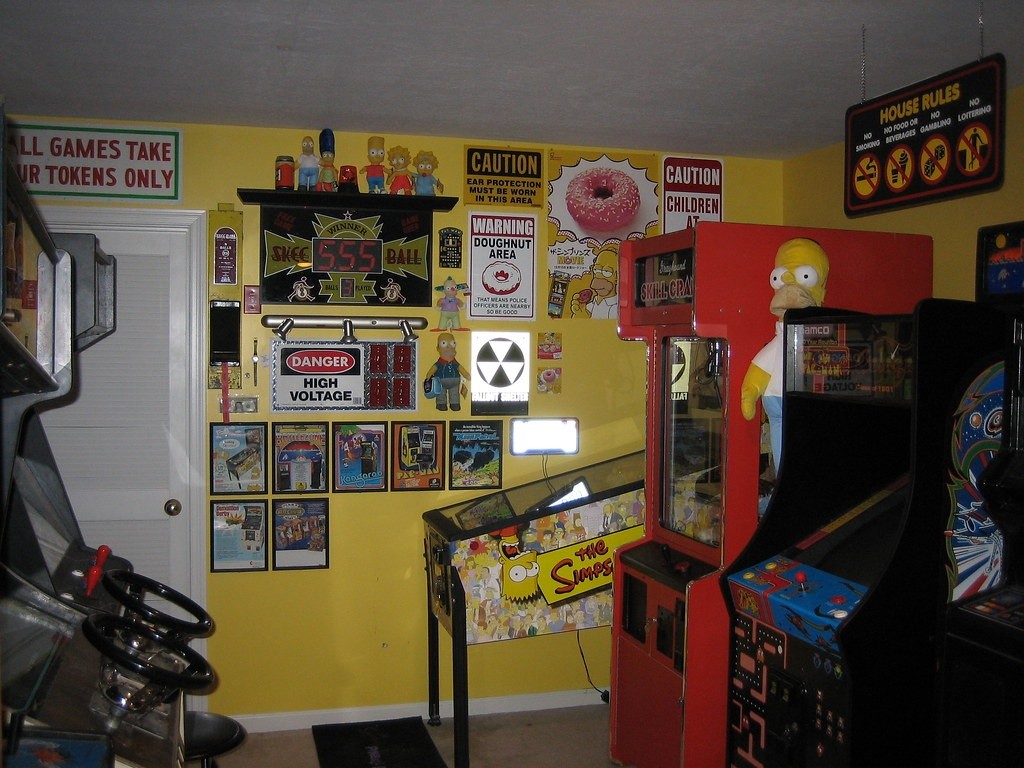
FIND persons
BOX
[423,333,470,412]
[293,129,338,192]
[359,136,444,197]
[430,276,470,333]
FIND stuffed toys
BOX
[741,238,831,519]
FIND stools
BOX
[181,710,246,768]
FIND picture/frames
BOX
[391,420,446,491]
[332,422,389,492]
[449,420,502,490]
[210,421,268,494]
[272,422,329,494]
[272,499,329,570]
[209,499,268,572]
